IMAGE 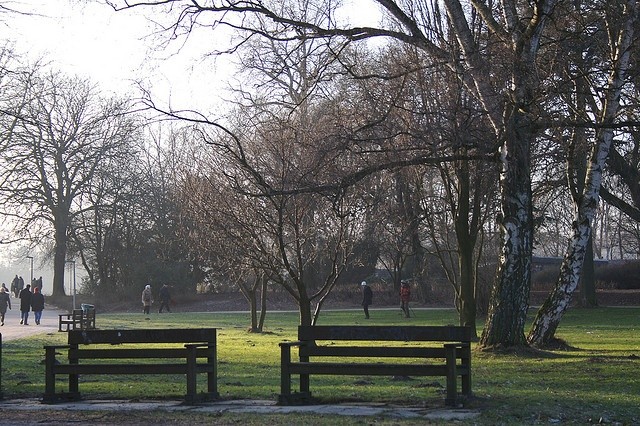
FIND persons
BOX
[142,284,154,316]
[12,274,18,299]
[31,287,45,325]
[158,282,172,313]
[400,280,412,319]
[0,287,12,327]
[18,284,33,326]
[31,277,36,293]
[360,281,373,320]
[16,276,24,298]
[36,276,43,294]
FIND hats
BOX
[400,280,406,284]
[0,288,4,292]
[34,288,38,292]
[361,281,366,285]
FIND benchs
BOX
[59,309,83,332]
[278,324,480,406]
[83,309,95,329]
[41,329,220,405]
